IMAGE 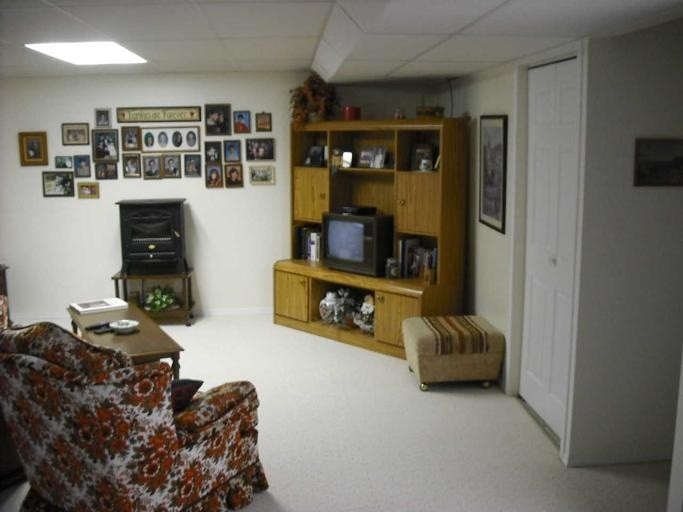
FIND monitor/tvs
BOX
[322,212,393,277]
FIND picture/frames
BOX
[476,110,508,237]
[356,146,387,169]
[630,136,682,188]
[16,101,277,201]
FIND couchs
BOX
[0,263,28,492]
[1,321,268,512]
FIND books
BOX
[300,227,320,263]
[397,237,436,279]
[69,296,130,315]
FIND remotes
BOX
[94,325,114,333]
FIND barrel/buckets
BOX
[339,105,361,121]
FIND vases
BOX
[305,110,328,123]
[318,292,337,324]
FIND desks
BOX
[111,267,195,328]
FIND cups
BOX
[418,157,432,172]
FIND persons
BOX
[27,112,273,195]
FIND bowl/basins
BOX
[110,319,137,334]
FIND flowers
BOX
[286,72,342,130]
[143,284,177,311]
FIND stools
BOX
[398,314,504,392]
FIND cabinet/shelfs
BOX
[272,116,469,362]
[114,197,189,275]
